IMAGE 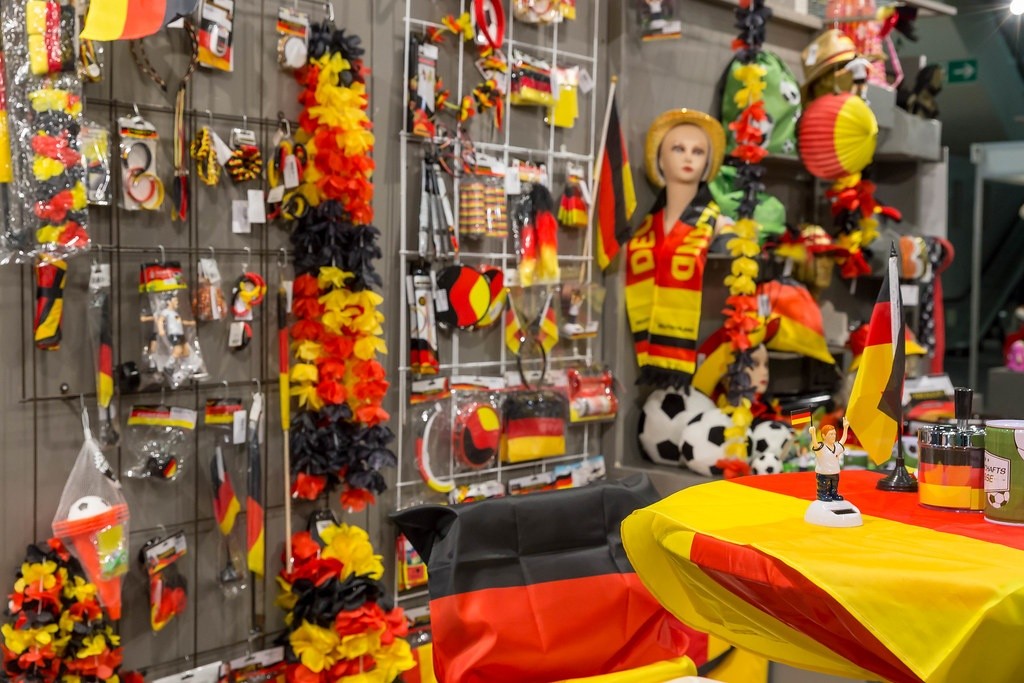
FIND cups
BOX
[984,418,1024,528]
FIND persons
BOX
[142,296,198,368]
[834,51,880,101]
[810,417,850,502]
[721,340,775,405]
[626,105,743,422]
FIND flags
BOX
[843,256,907,468]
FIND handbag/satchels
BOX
[504,336,571,461]
[717,44,805,157]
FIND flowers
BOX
[272,19,417,683]
[716,0,774,476]
[0,541,144,683]
[828,170,879,278]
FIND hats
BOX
[756,276,835,365]
[645,106,727,188]
[802,29,871,89]
[690,315,781,402]
[761,222,850,263]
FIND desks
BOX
[620,470,1024,683]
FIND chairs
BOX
[387,470,723,683]
